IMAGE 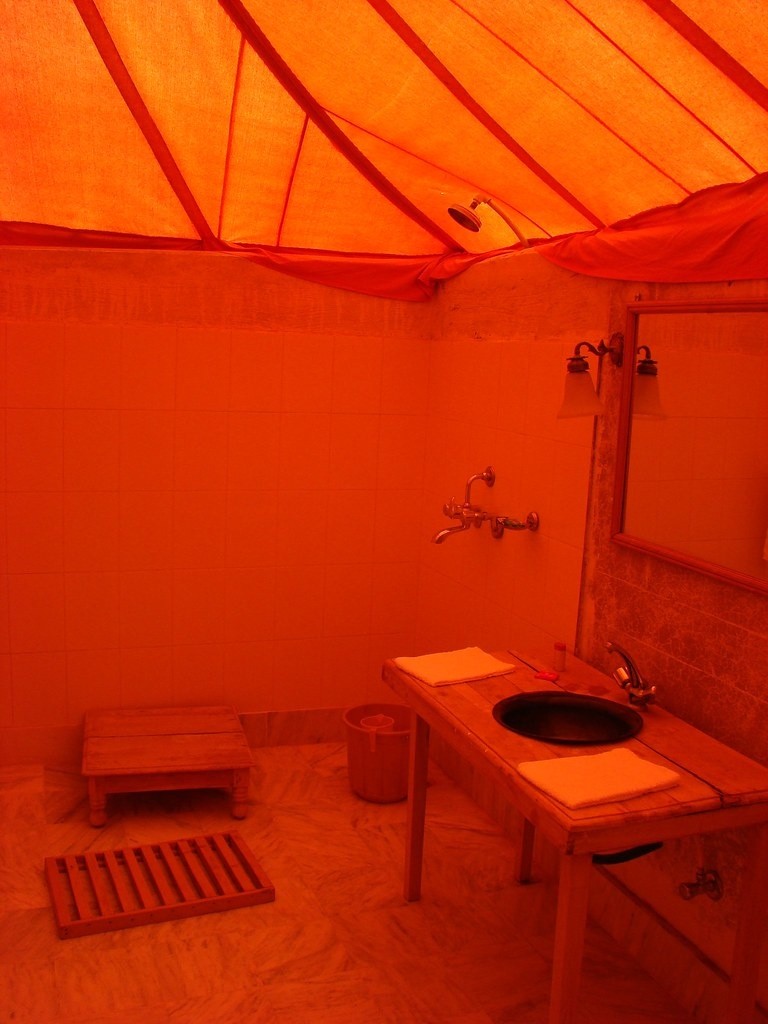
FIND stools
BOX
[83,706,254,827]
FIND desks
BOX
[382,641,768,1024]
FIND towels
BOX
[515,744,683,811]
[392,644,518,688]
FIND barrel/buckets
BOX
[343,703,412,804]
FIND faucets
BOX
[603,639,647,706]
[431,522,468,545]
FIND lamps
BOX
[636,344,664,416]
[556,332,624,419]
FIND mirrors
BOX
[608,296,768,596]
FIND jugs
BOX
[360,714,394,753]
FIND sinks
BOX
[490,684,645,747]
